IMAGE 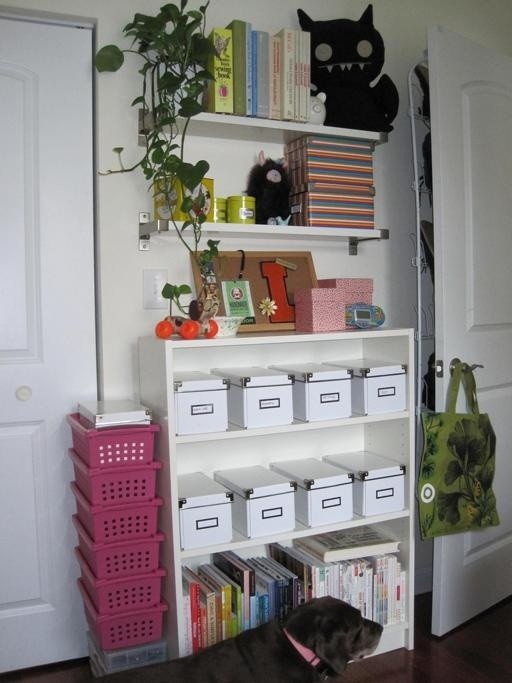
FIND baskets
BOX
[65,413,168,652]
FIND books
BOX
[182,523,406,655]
[203,19,311,123]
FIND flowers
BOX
[257,294,278,317]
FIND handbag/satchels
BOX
[417,362,500,539]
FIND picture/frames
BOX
[189,248,321,334]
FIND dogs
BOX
[80,595,383,683]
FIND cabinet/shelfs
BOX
[133,322,422,661]
[133,100,393,254]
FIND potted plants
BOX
[91,0,223,319]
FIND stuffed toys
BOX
[248,150,293,225]
[296,4,399,132]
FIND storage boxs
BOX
[291,283,349,335]
[319,277,375,310]
[288,181,378,231]
[210,363,298,432]
[267,358,357,425]
[155,174,219,227]
[272,455,356,531]
[320,355,410,418]
[278,132,375,185]
[176,469,238,553]
[323,449,410,520]
[175,367,232,438]
[86,629,168,677]
[77,396,152,462]
[211,462,299,540]
